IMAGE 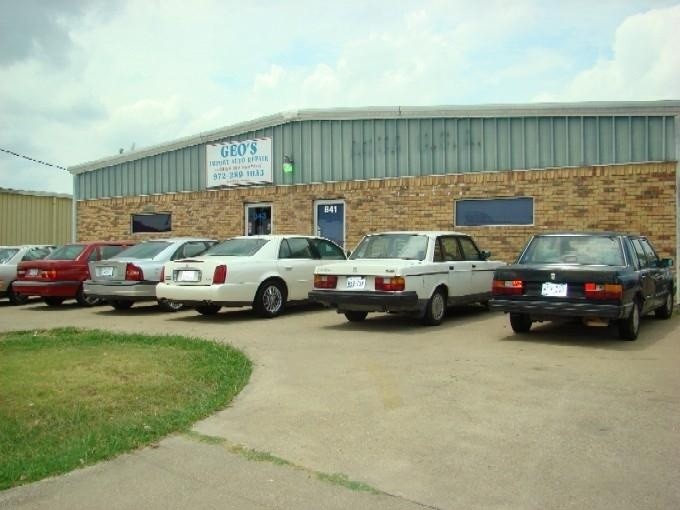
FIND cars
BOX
[308,231,509,325]
[82,239,218,312]
[0,245,58,303]
[12,242,136,306]
[156,234,352,317]
[488,232,676,340]
